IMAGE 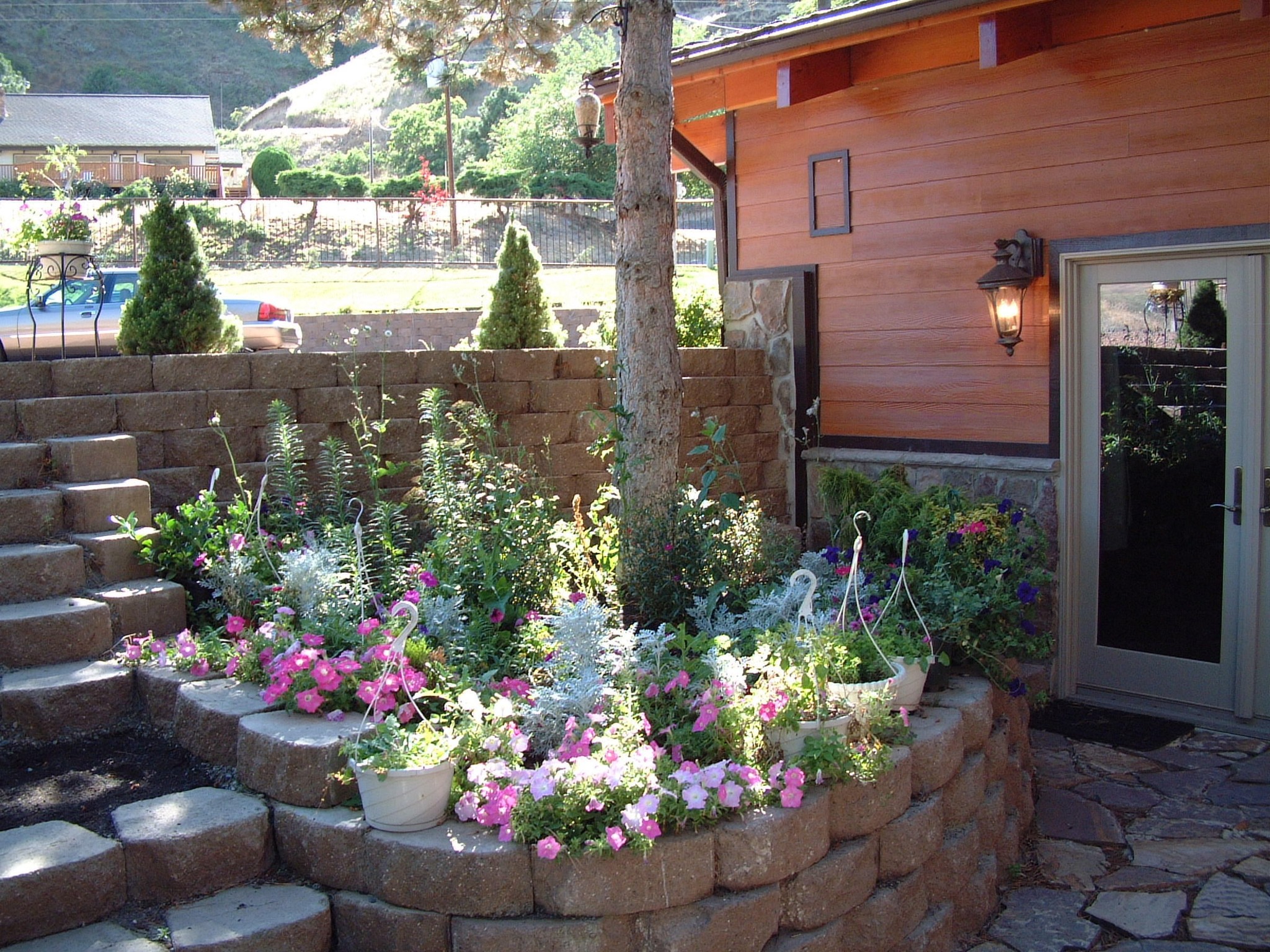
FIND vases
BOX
[351,742,454,833]
[37,239,94,277]
[830,656,903,729]
[764,700,853,766]
[893,651,936,712]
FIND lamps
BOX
[113,150,118,159]
[976,220,1046,363]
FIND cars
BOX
[0,266,303,362]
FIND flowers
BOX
[6,200,101,253]
[102,324,1050,859]
